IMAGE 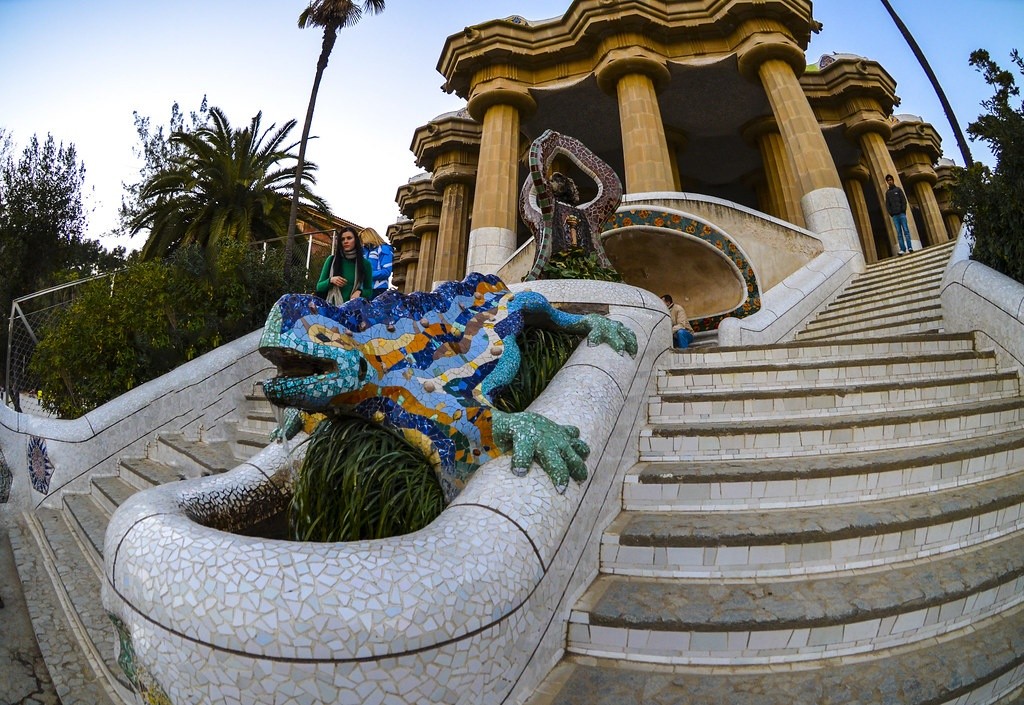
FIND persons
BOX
[359,228,393,300]
[314,227,372,308]
[884,174,914,256]
[660,294,695,349]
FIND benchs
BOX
[686,329,718,347]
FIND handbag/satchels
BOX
[324,284,345,307]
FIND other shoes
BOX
[909,248,914,252]
[897,250,905,255]
